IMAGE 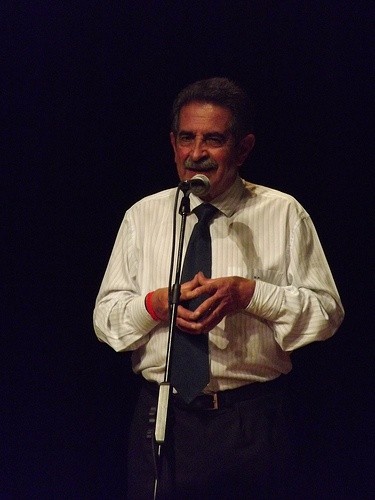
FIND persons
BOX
[94,76,346,500]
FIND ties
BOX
[168,203,221,403]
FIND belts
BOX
[168,371,294,412]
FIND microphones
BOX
[179,173,212,198]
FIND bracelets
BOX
[143,290,161,324]
